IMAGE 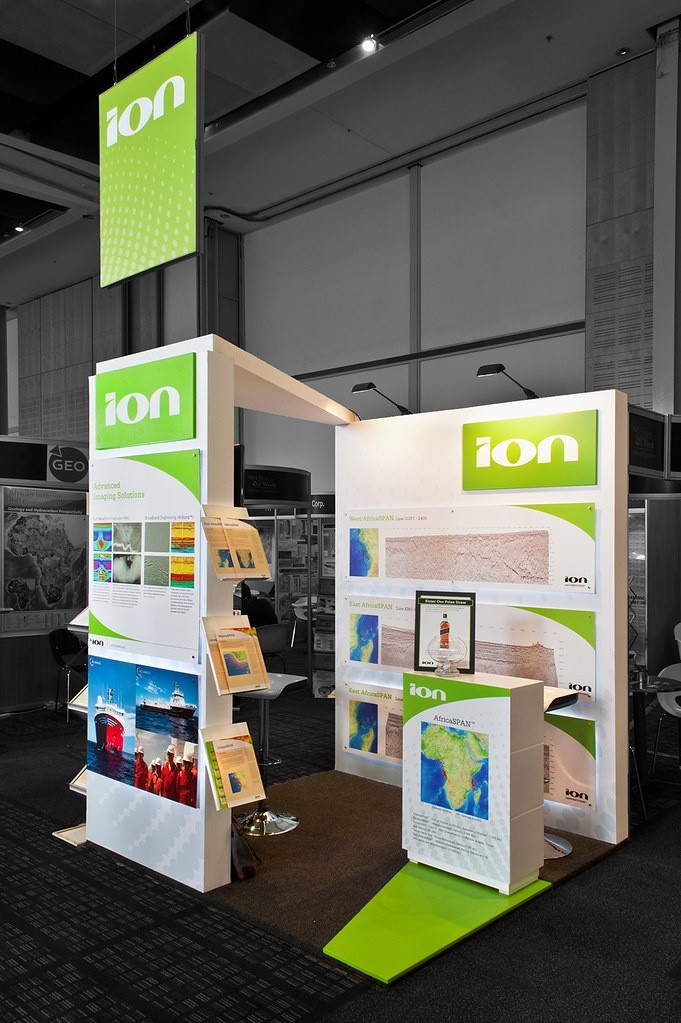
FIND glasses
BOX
[151,765,155,768]
[167,753,172,757]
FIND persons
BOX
[135,744,197,808]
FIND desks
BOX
[628,676,681,794]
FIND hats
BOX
[155,758,162,766]
[182,752,193,763]
[166,745,175,755]
[137,746,144,754]
[151,759,155,766]
[176,756,183,764]
[134,748,138,753]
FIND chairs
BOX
[257,624,288,674]
[648,663,681,777]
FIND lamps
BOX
[477,362,538,399]
[351,382,412,416]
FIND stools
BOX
[48,629,88,724]
[544,685,592,860]
[233,672,307,766]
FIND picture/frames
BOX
[414,590,476,675]
